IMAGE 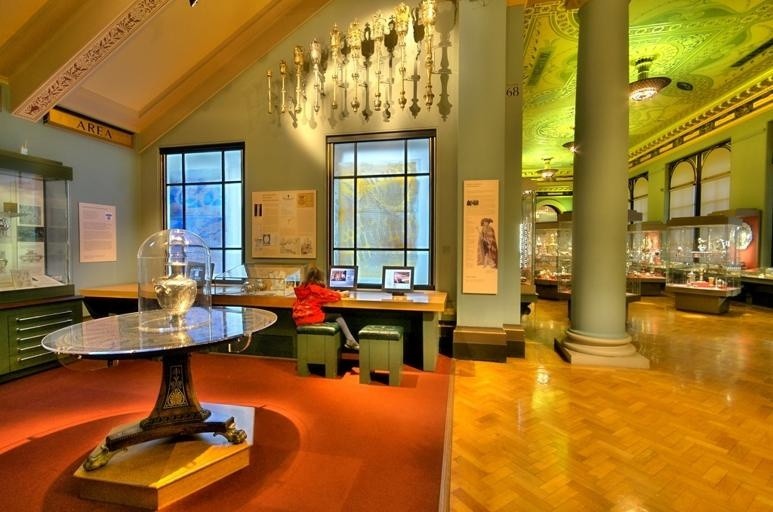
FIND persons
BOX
[291,266,360,351]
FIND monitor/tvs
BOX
[186,261,213,284]
[326,265,357,291]
[382,266,415,296]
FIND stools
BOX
[296,322,343,378]
[358,325,404,387]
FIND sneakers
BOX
[344,341,359,350]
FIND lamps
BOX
[536,159,559,178]
[189,0,197,8]
[629,57,671,102]
[560,126,575,151]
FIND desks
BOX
[40,304,276,470]
[80,284,447,373]
